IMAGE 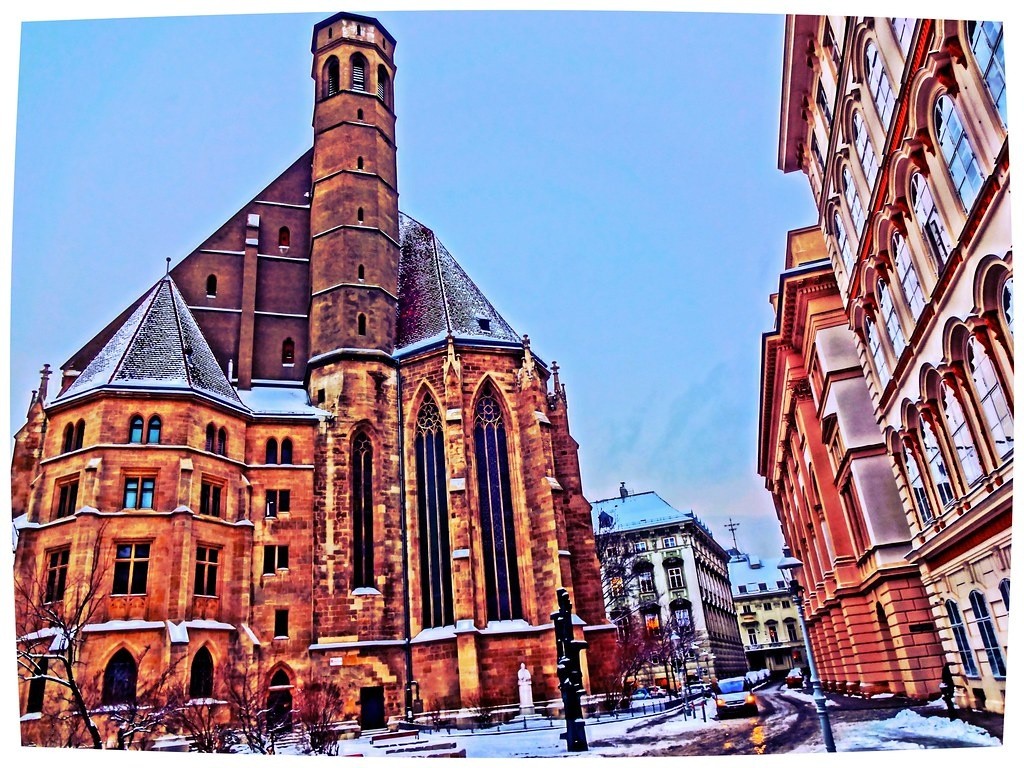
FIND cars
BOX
[631,686,667,699]
[784,667,804,689]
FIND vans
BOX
[715,677,758,719]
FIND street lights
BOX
[692,643,707,705]
[776,543,837,753]
[670,630,692,717]
[701,650,716,699]
